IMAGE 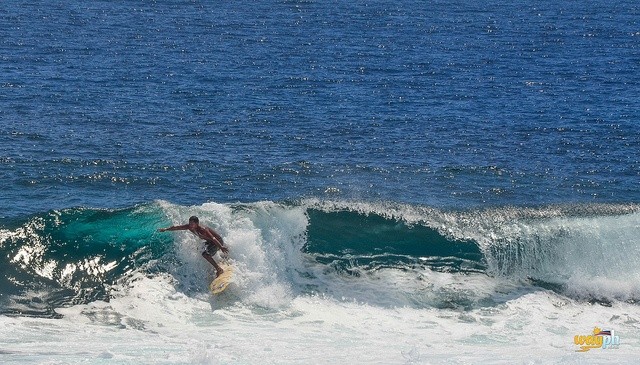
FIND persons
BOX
[157,216,229,277]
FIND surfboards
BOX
[211,261,233,293]
